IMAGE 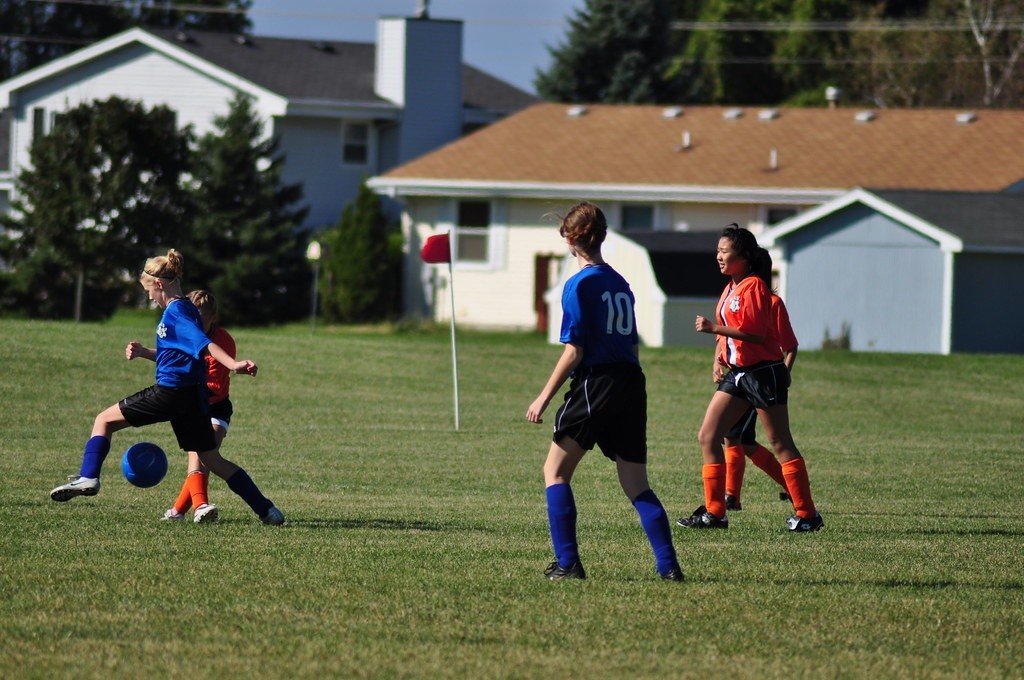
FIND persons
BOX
[50,249,287,525]
[678,223,825,531]
[527,202,684,581]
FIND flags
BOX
[422,235,448,261]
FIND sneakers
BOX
[260,506,285,526]
[661,569,684,581]
[50,474,100,502]
[194,504,218,524]
[724,493,742,511]
[159,508,185,521]
[544,561,586,581]
[786,511,824,534]
[676,505,729,530]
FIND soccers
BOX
[121,442,168,489]
